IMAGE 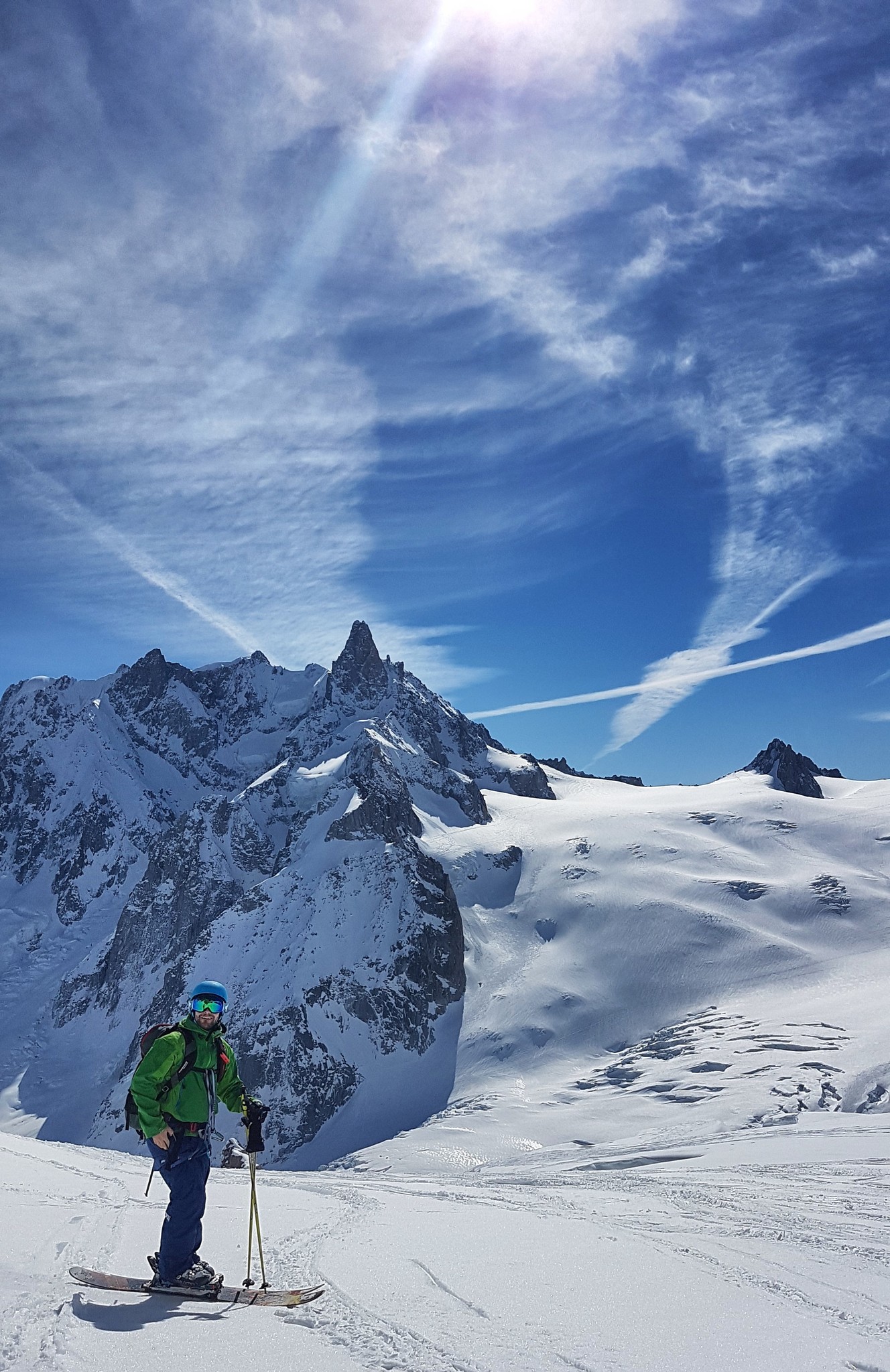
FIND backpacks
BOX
[124,1023,229,1131]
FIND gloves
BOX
[241,1100,271,1154]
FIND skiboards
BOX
[68,1266,326,1308]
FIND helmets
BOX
[192,981,228,1003]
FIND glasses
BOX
[193,998,225,1013]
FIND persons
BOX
[129,980,263,1286]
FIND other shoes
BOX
[195,1261,215,1277]
[155,1265,214,1288]
